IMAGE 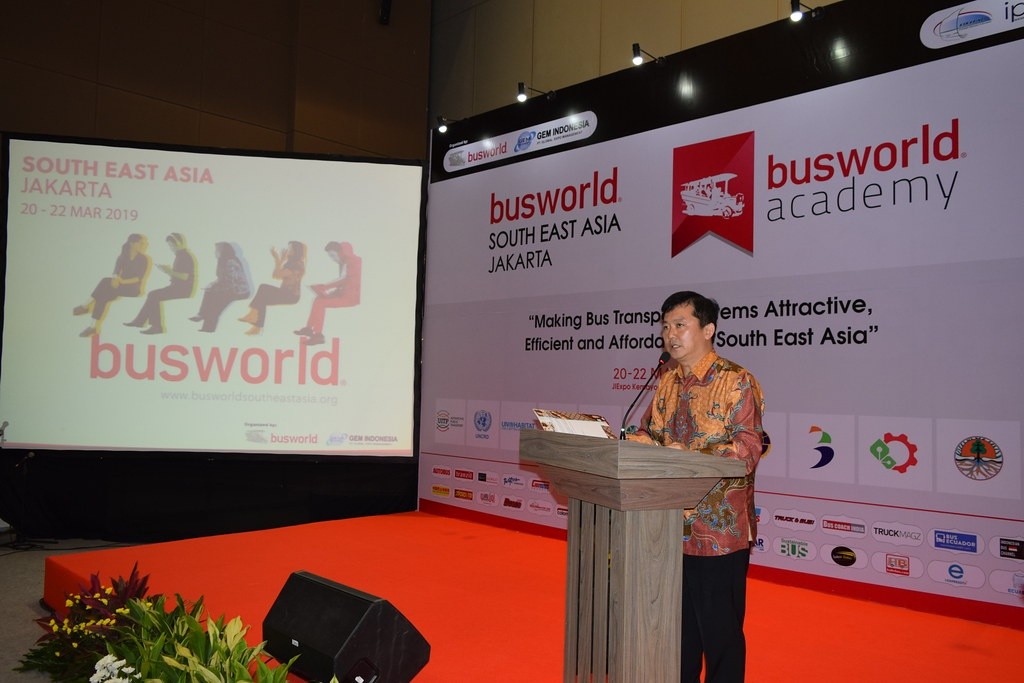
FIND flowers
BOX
[34,560,163,663]
[89,592,302,683]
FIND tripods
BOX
[0,517,58,547]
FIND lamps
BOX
[437,115,457,133]
[632,43,658,65]
[516,81,545,103]
[790,0,812,22]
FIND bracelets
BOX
[653,439,659,446]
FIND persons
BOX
[625,291,766,683]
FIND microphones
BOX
[0,421,8,435]
[620,351,671,440]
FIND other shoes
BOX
[189,317,205,322]
[123,320,144,327]
[294,327,325,344]
[79,327,96,337]
[73,305,88,314]
[140,327,163,334]
[198,329,216,332]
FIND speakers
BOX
[263,570,432,683]
